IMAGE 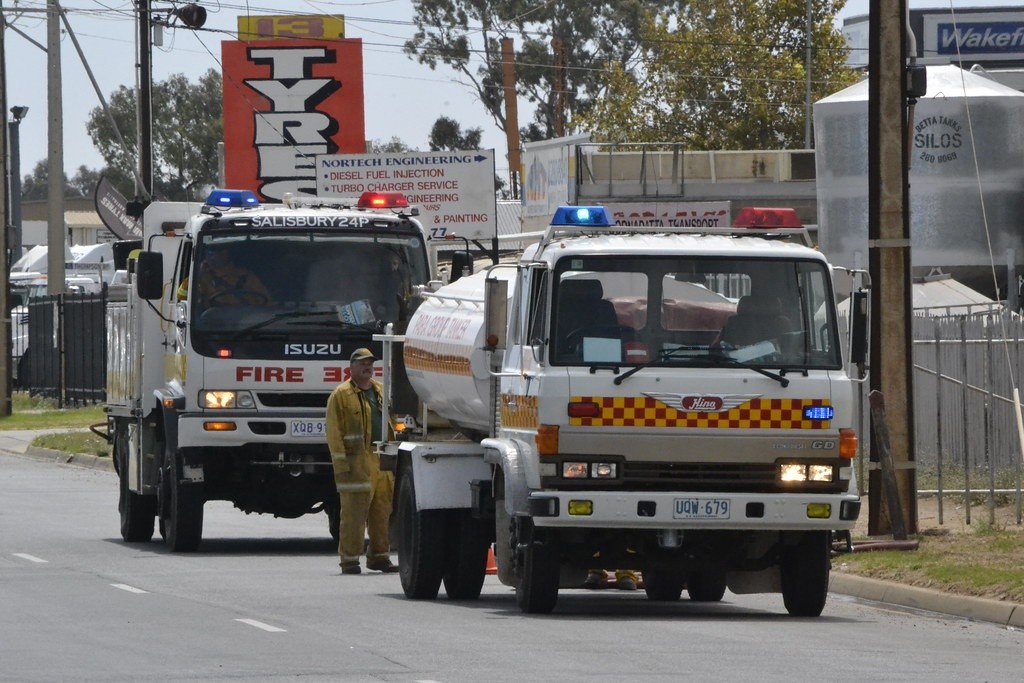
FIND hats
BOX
[350,348,378,363]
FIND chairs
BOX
[563,278,751,345]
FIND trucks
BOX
[9,240,116,383]
[376,203,875,620]
[88,187,474,557]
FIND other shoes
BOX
[618,577,637,590]
[342,561,361,574]
[584,574,608,588]
[366,560,399,572]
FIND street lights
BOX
[131,5,210,222]
[5,105,31,267]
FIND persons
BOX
[583,546,637,590]
[177,245,271,307]
[326,347,399,574]
[365,250,420,316]
